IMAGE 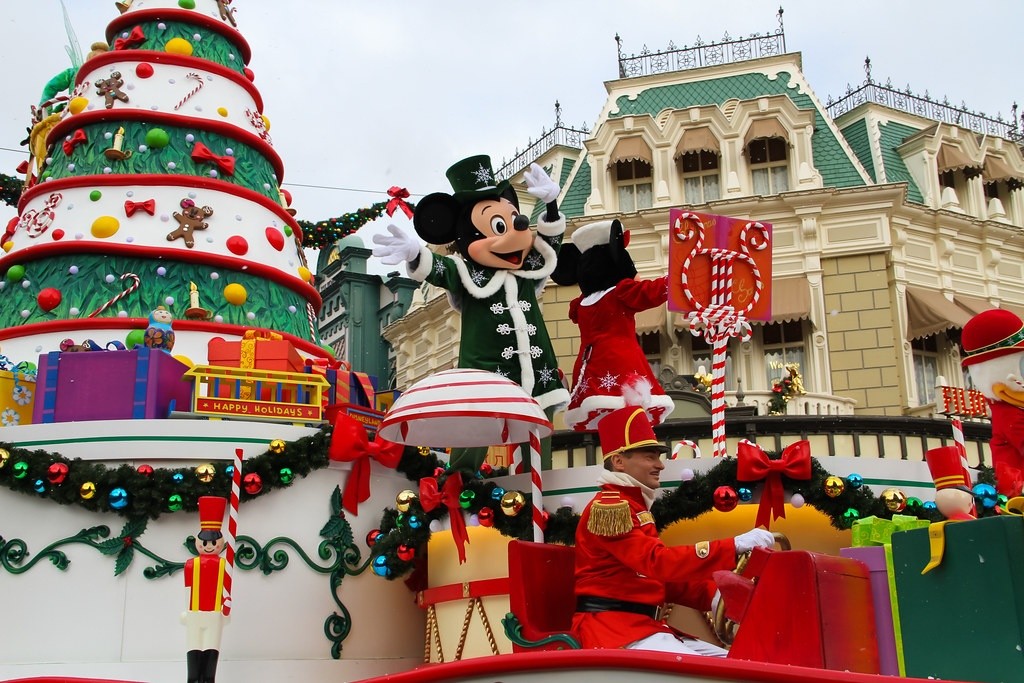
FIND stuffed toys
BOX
[371,155,570,415]
[548,219,675,430]
[960,309,1024,498]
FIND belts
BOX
[575,595,662,621]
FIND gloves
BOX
[734,528,775,553]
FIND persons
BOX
[184,496,227,683]
[570,405,775,659]
[925,445,977,521]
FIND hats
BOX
[597,405,671,462]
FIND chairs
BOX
[501,539,578,654]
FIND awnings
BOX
[906,287,1024,341]
[607,137,652,165]
[674,127,720,161]
[983,156,1024,192]
[634,277,811,338]
[936,144,982,181]
[743,118,790,151]
